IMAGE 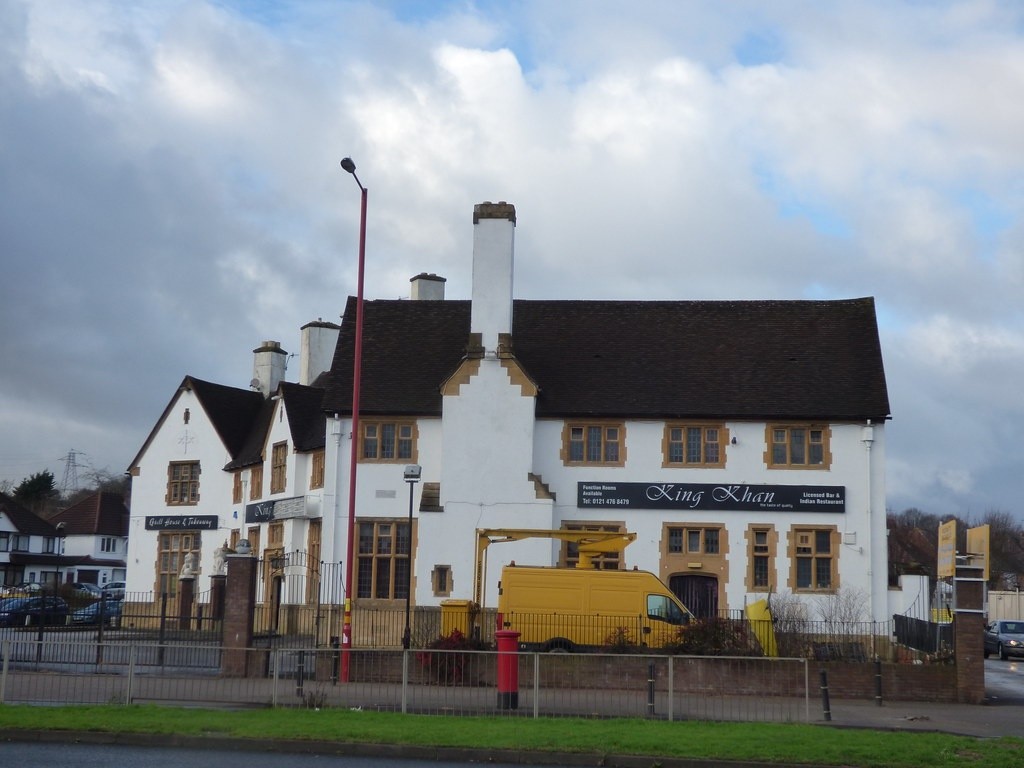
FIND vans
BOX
[473,529,701,658]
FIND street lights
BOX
[55,521,65,599]
[402,463,422,650]
[338,155,367,682]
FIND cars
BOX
[100,581,127,600]
[0,583,41,599]
[0,599,68,628]
[984,619,1024,661]
[71,583,103,599]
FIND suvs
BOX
[72,601,123,626]
